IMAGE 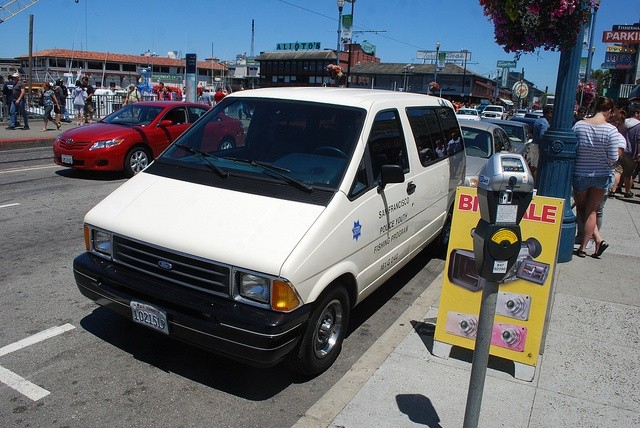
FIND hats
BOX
[12,72,19,77]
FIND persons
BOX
[572,95,621,258]
[5,73,29,130]
[165,90,172,101]
[82,77,96,125]
[3,75,15,116]
[0,75,5,121]
[199,89,213,107]
[447,131,464,155]
[573,106,589,129]
[196,88,205,103]
[52,81,63,125]
[435,139,446,158]
[40,81,62,131]
[59,79,68,122]
[214,88,225,105]
[573,105,629,250]
[238,100,254,121]
[122,83,141,110]
[181,87,186,101]
[607,102,640,200]
[157,82,166,101]
[529,104,555,190]
[72,80,84,127]
[452,98,465,113]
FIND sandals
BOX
[576,249,586,257]
[591,239,609,257]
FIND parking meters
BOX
[448,151,550,427]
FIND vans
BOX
[543,95,555,111]
[73,87,467,376]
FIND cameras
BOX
[444,310,479,341]
[495,293,534,320]
[447,227,551,292]
[492,322,528,353]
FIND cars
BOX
[456,117,514,183]
[534,109,544,115]
[510,117,537,135]
[483,104,506,118]
[454,108,481,121]
[53,100,245,177]
[482,117,534,166]
[524,113,541,119]
